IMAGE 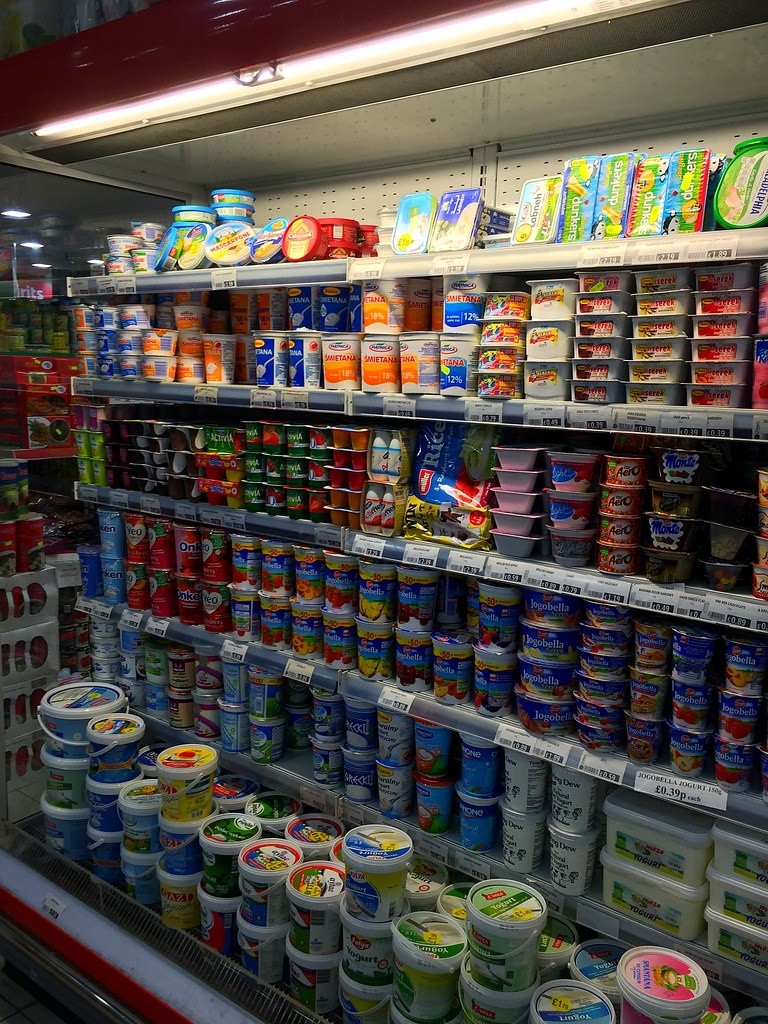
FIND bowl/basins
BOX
[488,446,768,602]
[73,282,209,384]
[101,189,397,273]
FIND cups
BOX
[69,402,372,530]
[622,617,768,805]
[203,273,489,397]
[85,615,311,766]
[71,268,579,402]
[309,682,593,897]
[0,458,47,782]
[76,507,519,718]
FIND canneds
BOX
[1,260,766,896]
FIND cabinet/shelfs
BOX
[0,226,768,1024]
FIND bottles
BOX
[58,586,92,680]
[364,430,400,536]
[0,298,72,354]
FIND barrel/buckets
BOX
[345,818,768,1024]
[35,681,341,1015]
